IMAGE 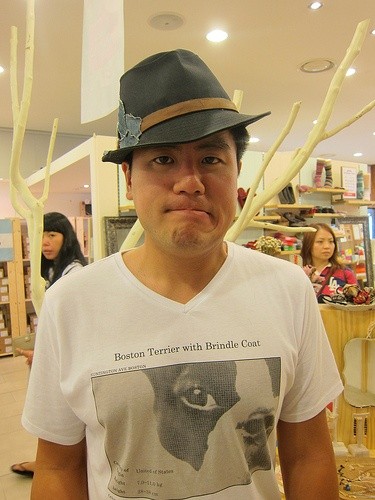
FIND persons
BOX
[21,50,347,500]
[12,212,88,475]
[298,224,359,413]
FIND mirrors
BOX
[331,216,374,288]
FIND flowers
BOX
[254,237,282,255]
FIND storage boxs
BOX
[0,235,39,354]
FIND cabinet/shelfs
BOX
[232,185,375,256]
[0,216,94,356]
